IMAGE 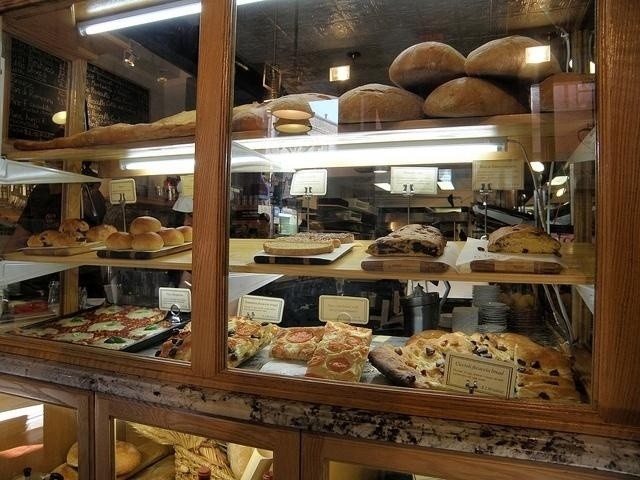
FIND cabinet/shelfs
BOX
[0,0,639,480]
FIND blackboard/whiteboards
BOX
[8,37,149,141]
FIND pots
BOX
[163,186,177,201]
[398,291,440,337]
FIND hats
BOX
[172,181,195,212]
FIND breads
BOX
[67,440,142,476]
[487,223,562,255]
[12,303,193,363]
[263,232,354,257]
[26,215,193,251]
[365,223,448,258]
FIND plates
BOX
[439,283,544,339]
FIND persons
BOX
[171,210,194,295]
[5,160,106,298]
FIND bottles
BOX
[47,281,61,316]
